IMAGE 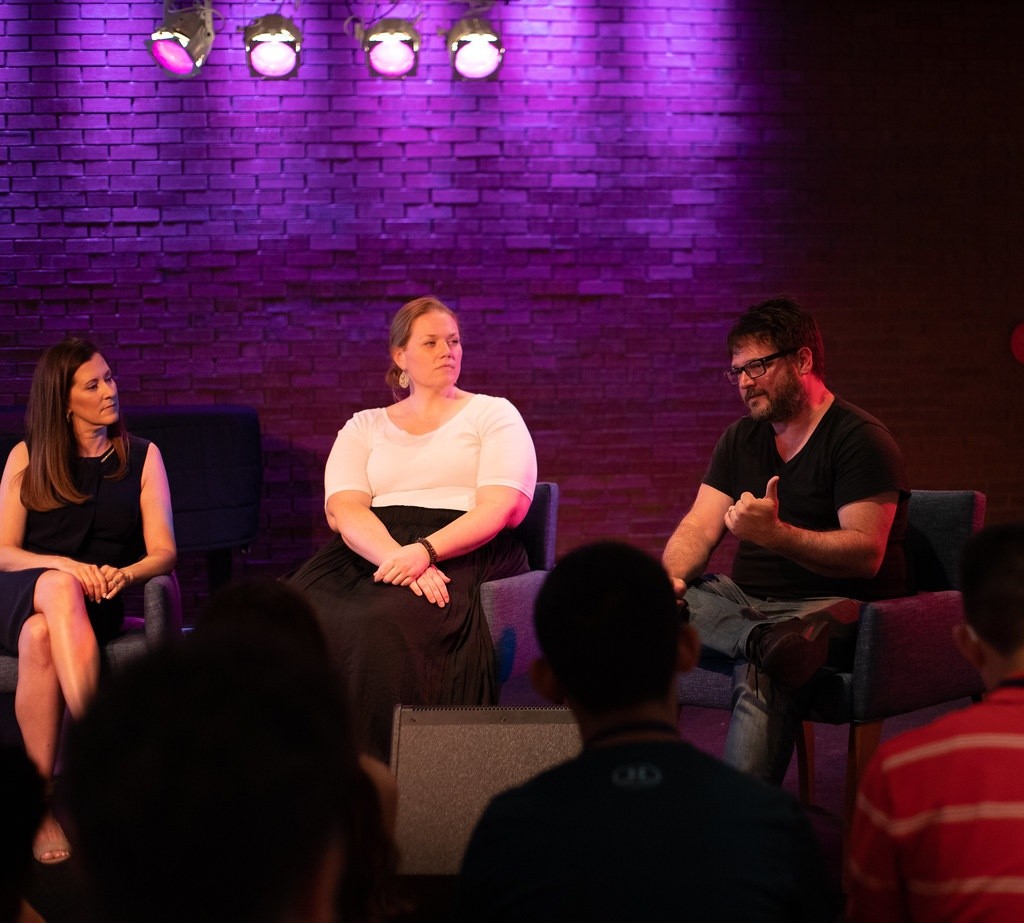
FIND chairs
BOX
[675,489,986,835]
[478,480,559,705]
[0,569,183,744]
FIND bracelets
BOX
[416,538,437,563]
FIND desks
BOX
[124,408,264,590]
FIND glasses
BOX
[724,346,800,386]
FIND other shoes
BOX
[745,619,833,699]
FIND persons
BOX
[284,298,538,761]
[0,337,178,864]
[661,297,915,796]
[462,541,846,923]
[0,586,398,922]
[847,520,1024,923]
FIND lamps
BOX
[360,18,421,81]
[236,12,304,81]
[438,16,506,82]
[143,0,215,81]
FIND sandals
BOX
[34,839,71,863]
